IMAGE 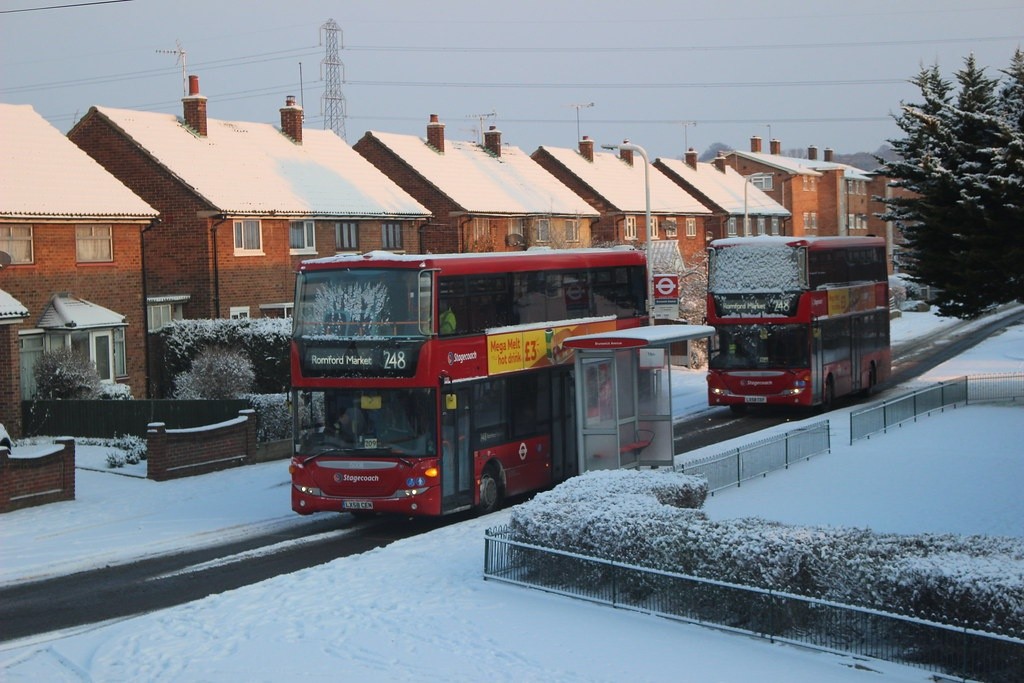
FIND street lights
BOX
[744,172,764,236]
[618,144,655,327]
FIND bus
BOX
[286,245,655,520]
[703,234,892,414]
